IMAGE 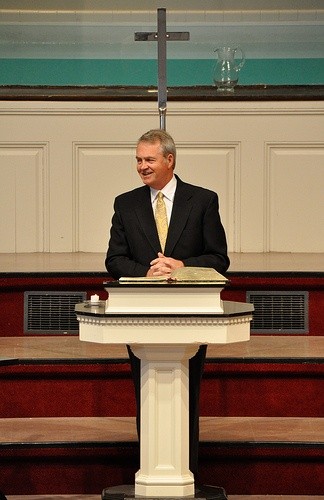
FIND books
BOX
[118,267,229,284]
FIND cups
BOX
[91,306,98,314]
[91,295,99,302]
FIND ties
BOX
[155,192,168,255]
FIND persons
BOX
[105,129,231,484]
